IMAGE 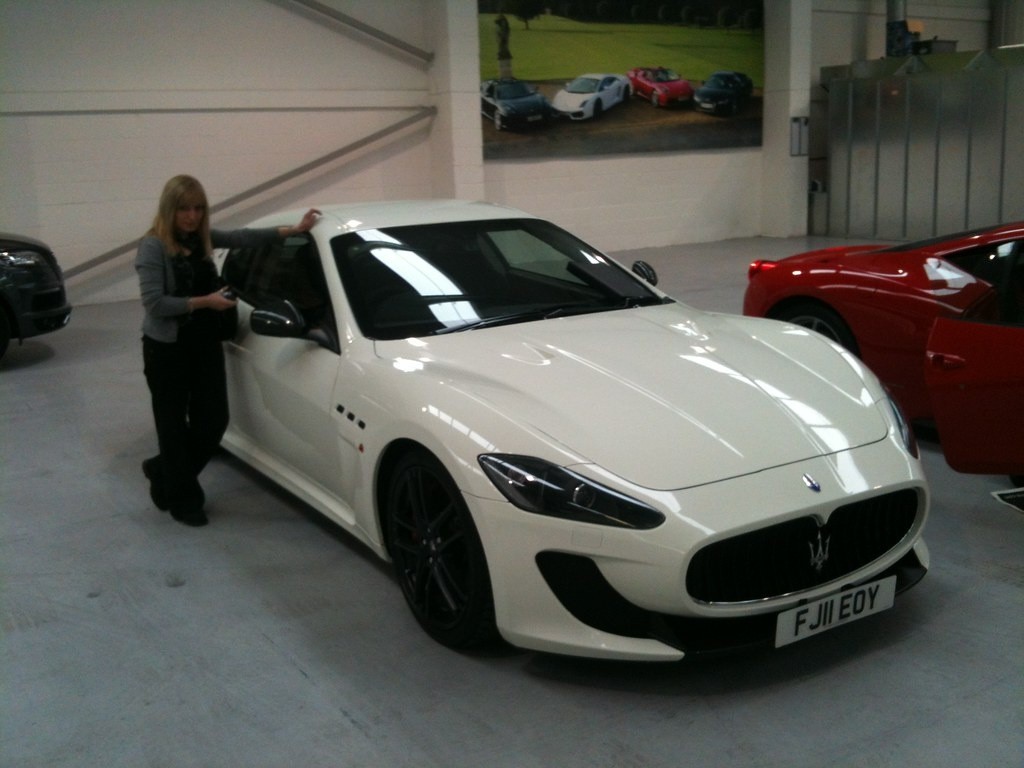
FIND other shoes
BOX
[141,455,174,510]
[170,506,208,527]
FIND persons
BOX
[134,174,323,527]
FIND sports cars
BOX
[211,199,932,674]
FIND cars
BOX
[550,72,630,121]
[692,68,753,115]
[481,76,551,130]
[0,230,74,368]
[744,219,1024,507]
[628,66,694,112]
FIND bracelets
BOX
[289,225,292,235]
